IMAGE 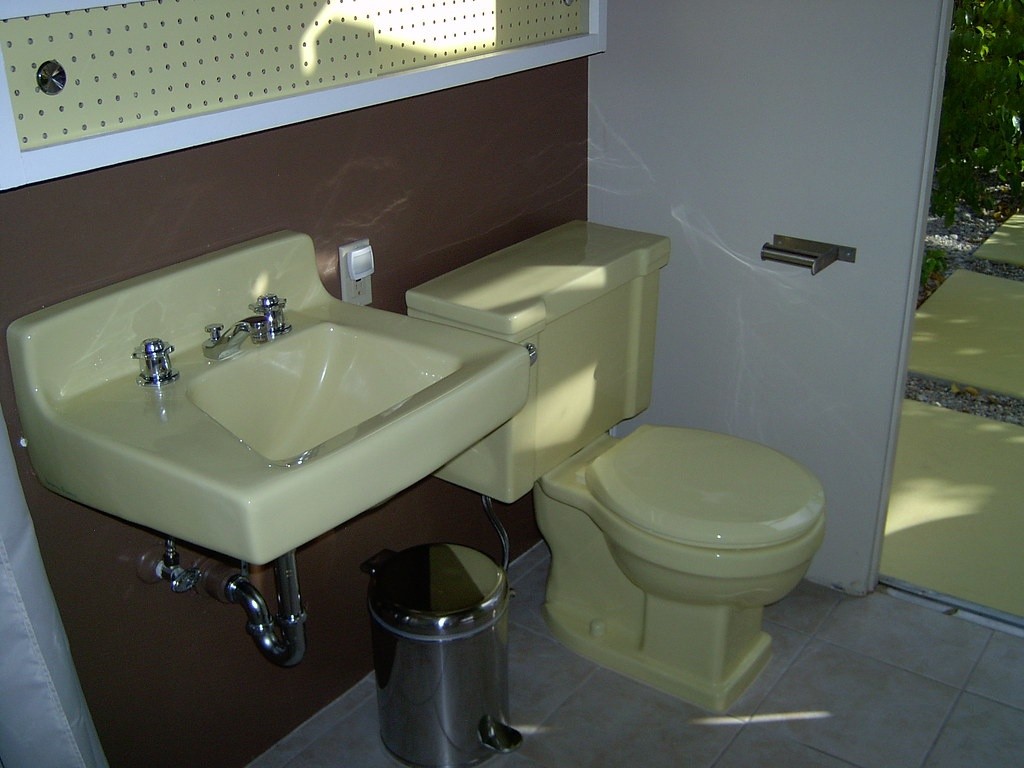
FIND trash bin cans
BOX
[359,542,526,768]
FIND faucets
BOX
[202,316,269,360]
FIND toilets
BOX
[407,220,824,708]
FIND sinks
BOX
[7,230,532,567]
